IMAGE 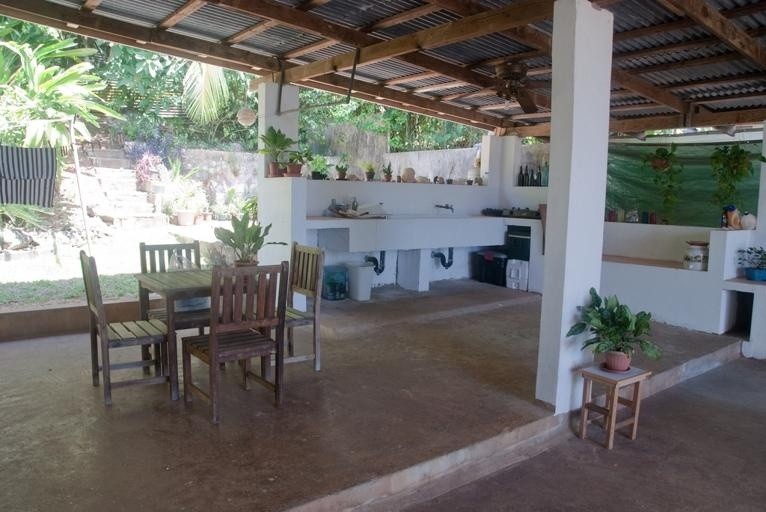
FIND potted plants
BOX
[213,211,288,281]
[565,286,663,375]
[640,141,682,213]
[705,144,756,206]
[735,245,766,282]
[255,125,395,182]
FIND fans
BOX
[468,60,552,114]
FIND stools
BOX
[578,363,652,451]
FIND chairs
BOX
[140,242,205,381]
[182,261,288,425]
[242,241,324,372]
[79,250,169,407]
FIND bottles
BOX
[518,160,549,187]
[608,207,625,222]
[740,210,756,230]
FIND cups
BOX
[641,208,656,224]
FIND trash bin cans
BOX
[323,261,376,302]
